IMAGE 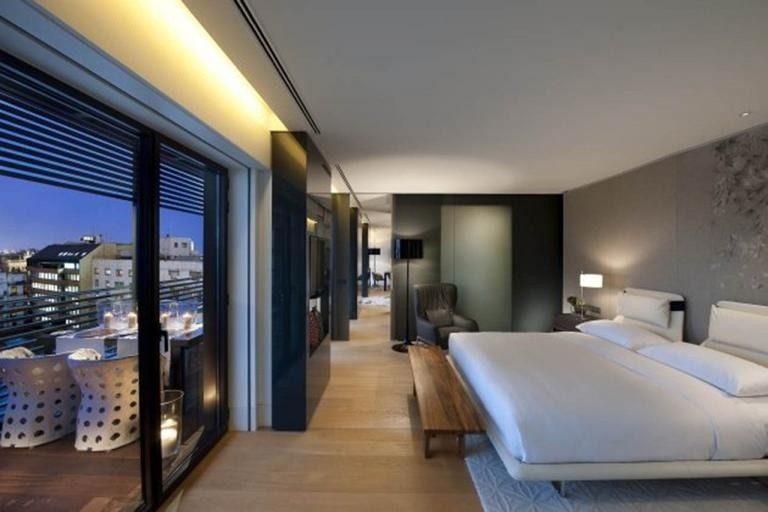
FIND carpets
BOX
[455,427,767,512]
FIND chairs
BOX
[66,350,167,452]
[0,351,80,450]
[412,283,479,349]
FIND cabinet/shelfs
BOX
[308,332,330,422]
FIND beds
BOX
[442,285,767,495]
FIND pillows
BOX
[574,317,672,353]
[69,347,101,361]
[0,347,35,359]
[615,292,671,329]
[635,340,767,397]
[425,309,453,327]
[707,302,767,354]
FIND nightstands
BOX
[549,311,600,332]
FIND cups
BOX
[103,311,191,330]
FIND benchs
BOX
[406,344,487,459]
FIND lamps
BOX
[576,273,603,322]
[368,247,380,288]
[391,239,423,353]
